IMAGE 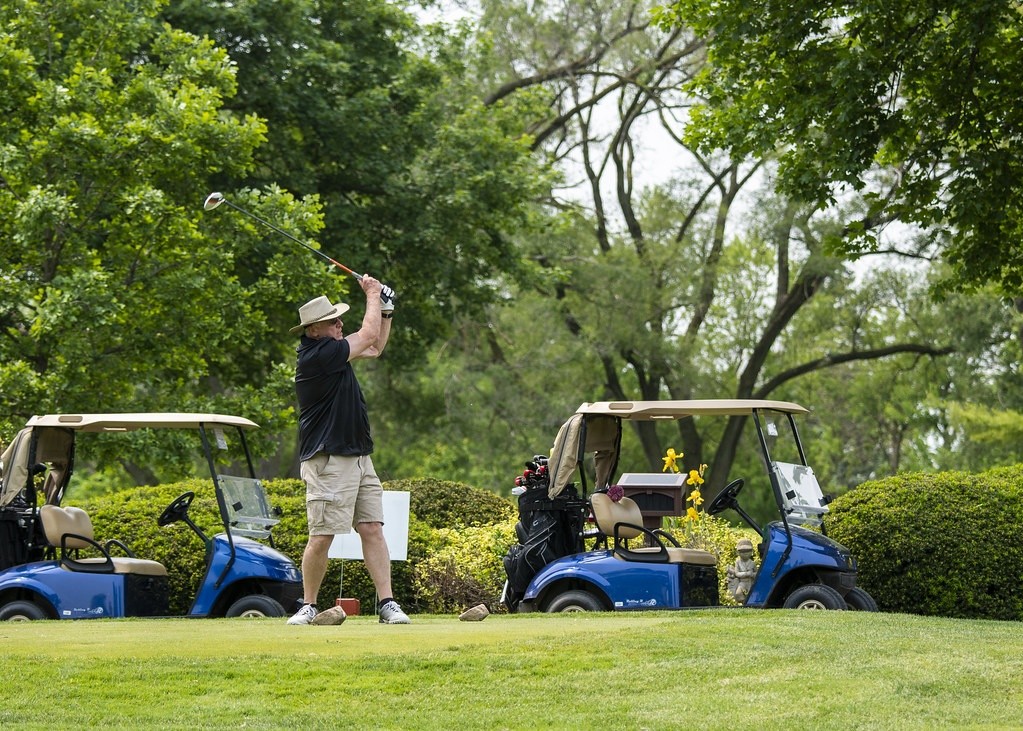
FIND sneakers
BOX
[287,604,317,625]
[379,601,412,624]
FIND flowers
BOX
[608,485,624,502]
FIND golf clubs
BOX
[203,191,363,280]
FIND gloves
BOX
[380,284,396,311]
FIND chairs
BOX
[589,490,719,566]
[38,503,170,578]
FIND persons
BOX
[287,274,412,624]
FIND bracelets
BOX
[381,312,393,318]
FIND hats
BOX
[288,295,350,333]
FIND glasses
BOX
[329,317,340,325]
